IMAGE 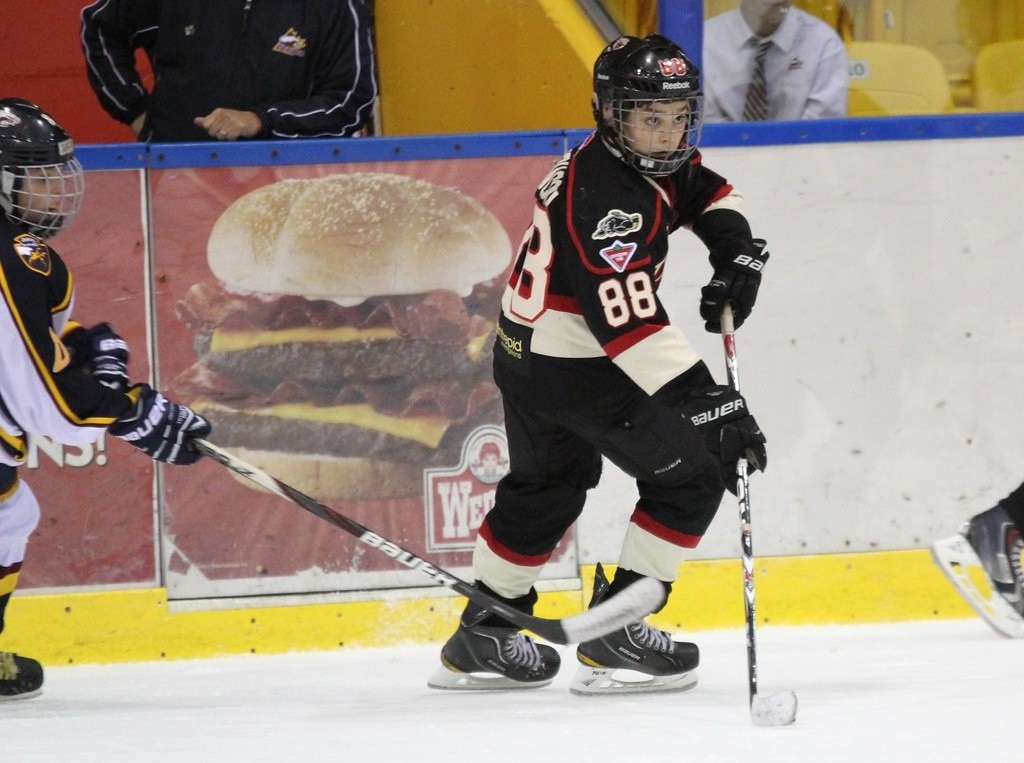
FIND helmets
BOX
[0,97,73,166]
[593,33,704,110]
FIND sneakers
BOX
[0,651,43,695]
[568,562,701,698]
[931,503,1024,640]
[426,579,561,693]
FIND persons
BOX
[702,0,849,122]
[80,0,380,144]
[0,96,212,706]
[429,33,769,697]
[932,482,1024,641]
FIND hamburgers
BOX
[164,172,513,500]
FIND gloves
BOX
[698,238,770,335]
[678,385,768,497]
[86,332,130,391]
[107,383,212,466]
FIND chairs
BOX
[606,0,1024,121]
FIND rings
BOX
[218,130,226,136]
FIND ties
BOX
[740,37,774,122]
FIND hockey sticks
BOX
[186,433,666,647]
[719,303,799,728]
